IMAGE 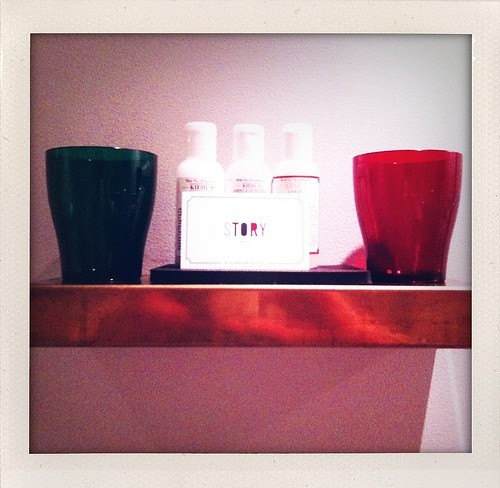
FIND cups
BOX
[352,149,464,285]
[45,145,159,283]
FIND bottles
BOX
[175,122,224,266]
[225,124,271,193]
[269,121,322,270]
